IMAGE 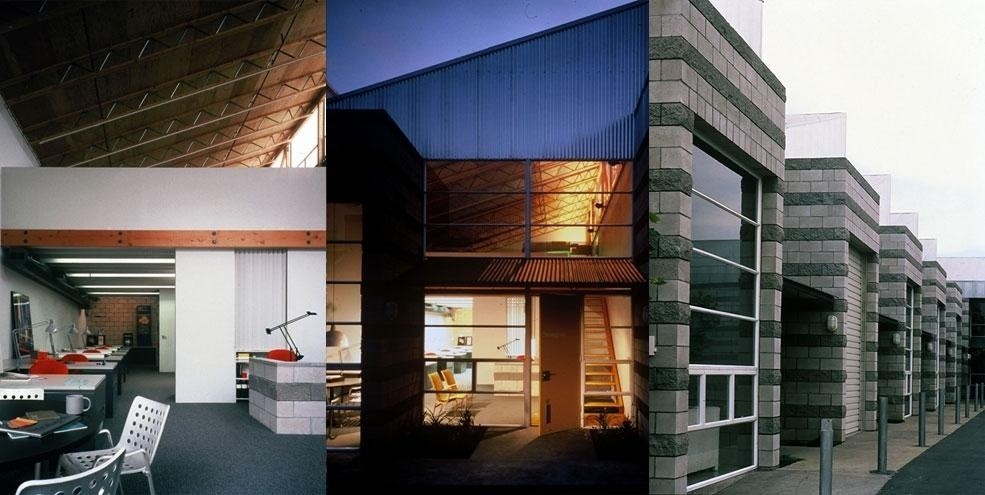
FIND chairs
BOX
[54,395,171,495]
[327,369,362,446]
[15,448,126,495]
[269,350,298,363]
[428,369,466,417]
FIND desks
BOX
[0,344,134,479]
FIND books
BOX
[0,410,88,439]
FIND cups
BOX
[65,394,91,415]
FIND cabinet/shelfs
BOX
[236,352,269,401]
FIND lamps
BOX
[81,326,92,349]
[53,323,79,354]
[267,311,317,362]
[497,338,519,360]
[12,319,56,360]
[325,203,350,348]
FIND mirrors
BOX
[12,292,34,359]
